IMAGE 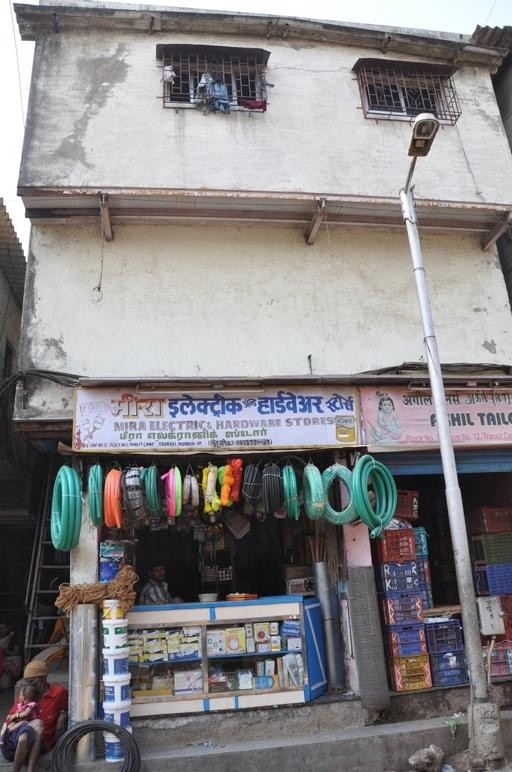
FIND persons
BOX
[138,562,183,605]
[368,392,401,443]
[0,659,68,772]
[8,684,37,724]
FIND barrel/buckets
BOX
[102,618,128,648]
[102,676,133,710]
[102,600,125,620]
[104,709,131,733]
[103,730,126,763]
[102,648,130,676]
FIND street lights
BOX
[396,110,510,770]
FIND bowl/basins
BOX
[198,593,218,602]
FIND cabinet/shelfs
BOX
[129,593,307,717]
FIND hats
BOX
[23,660,48,679]
